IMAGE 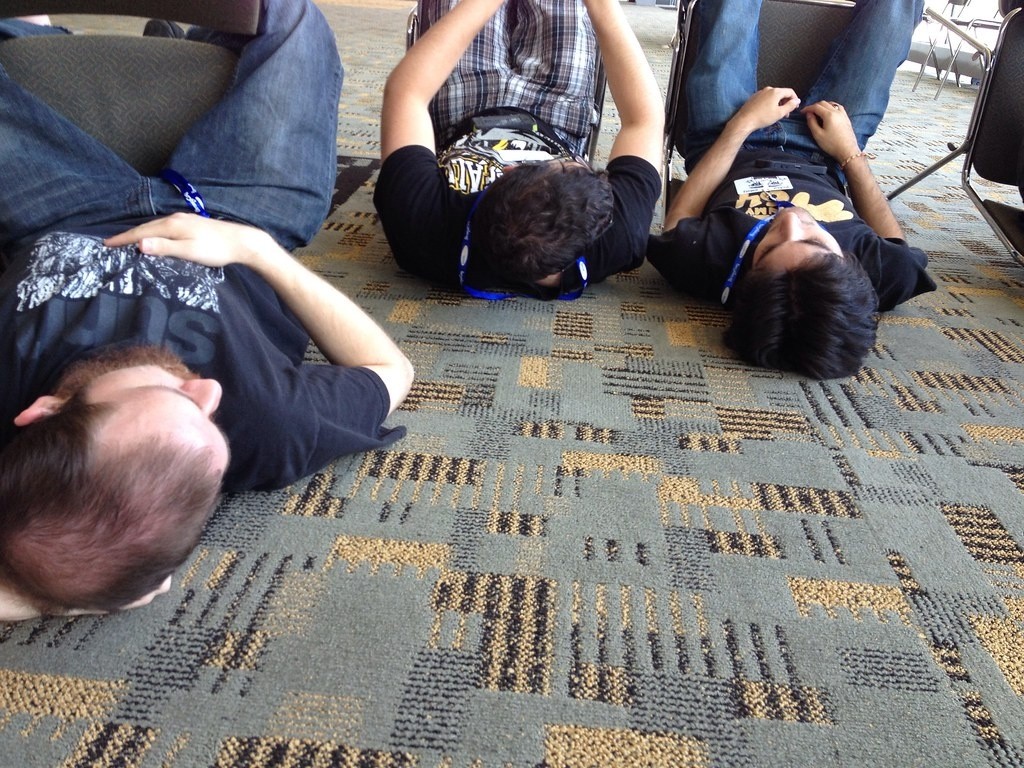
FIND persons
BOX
[371,0,667,301]
[645,0,938,379]
[0,0,416,623]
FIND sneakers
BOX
[142,17,186,41]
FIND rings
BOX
[832,104,838,109]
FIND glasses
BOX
[537,153,597,176]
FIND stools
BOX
[653,0,865,228]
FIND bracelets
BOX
[839,152,869,171]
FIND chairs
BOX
[888,0,1024,264]
[0,0,262,181]
